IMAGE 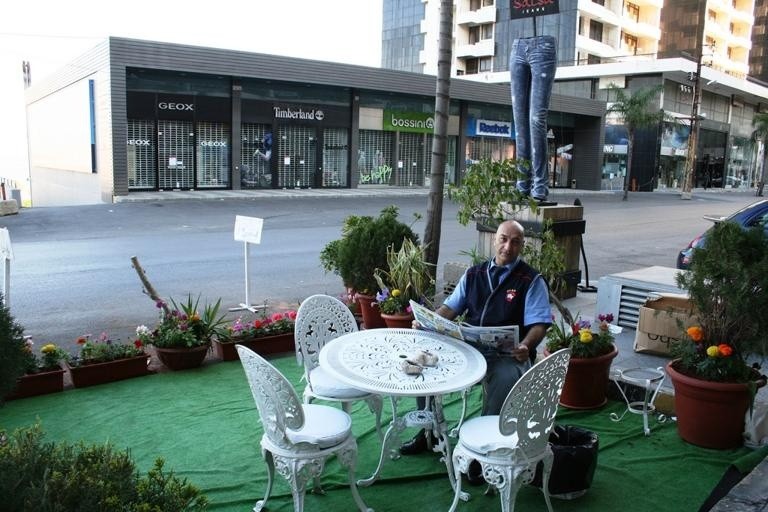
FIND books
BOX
[409,299,520,357]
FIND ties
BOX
[492,266,507,289]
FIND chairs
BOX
[235,342,376,512]
[448,347,575,512]
[293,293,401,462]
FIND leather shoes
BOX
[467,460,485,485]
[399,428,444,455]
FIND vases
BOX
[64,351,151,389]
[664,357,767,448]
[380,312,415,328]
[152,343,211,370]
[358,294,385,329]
[6,366,66,400]
[212,332,294,361]
[542,343,619,409]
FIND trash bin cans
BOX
[539,424,599,501]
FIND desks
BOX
[319,328,489,500]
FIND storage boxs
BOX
[633,292,720,358]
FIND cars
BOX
[674,198,768,272]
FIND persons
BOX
[398,220,553,486]
[254,128,273,162]
[509,35,558,203]
[357,149,387,184]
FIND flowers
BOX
[675,219,768,386]
[221,309,296,339]
[22,343,62,374]
[62,332,146,368]
[371,235,436,315]
[544,311,615,358]
[318,204,425,304]
[134,293,231,347]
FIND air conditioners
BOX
[593,265,762,331]
